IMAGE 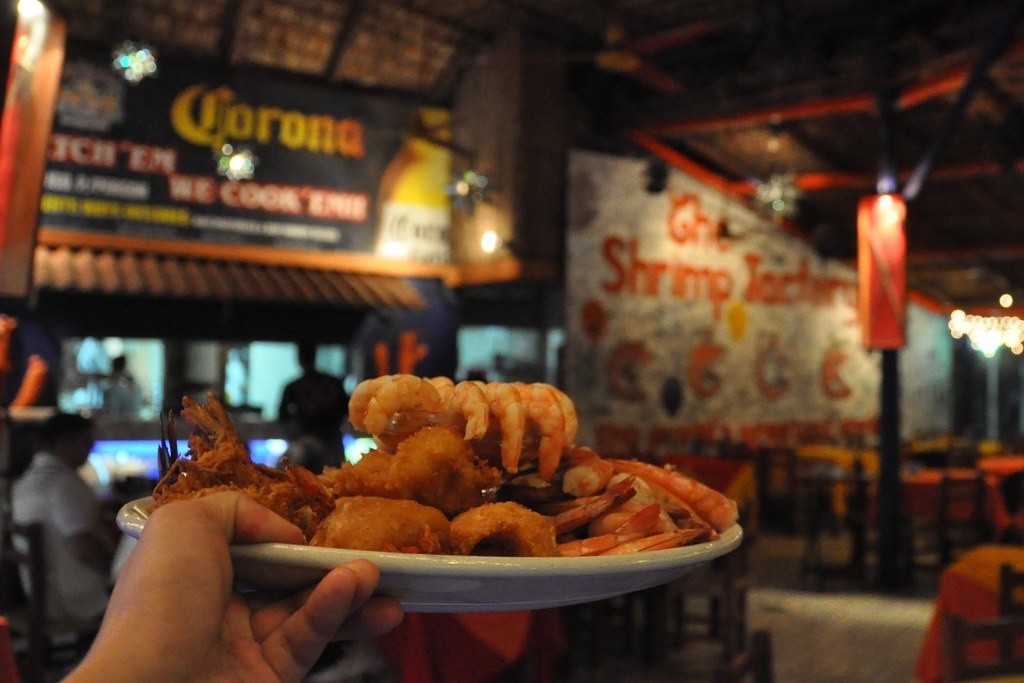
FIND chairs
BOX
[1,503,101,683]
[916,545,1023,683]
[559,438,1024,683]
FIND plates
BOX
[116,490,742,616]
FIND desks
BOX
[734,444,882,525]
[869,467,991,525]
[387,606,562,682]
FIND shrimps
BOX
[151,371,739,558]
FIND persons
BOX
[0,291,350,634]
[60,491,401,683]
